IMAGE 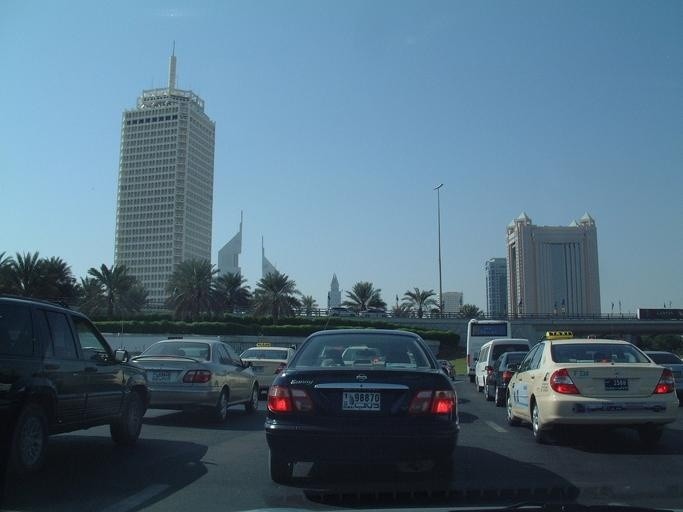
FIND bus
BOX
[466,318,512,383]
[637,308,683,320]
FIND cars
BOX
[127,338,261,424]
[437,359,456,381]
[264,329,461,482]
[239,342,296,390]
[320,345,417,368]
[472,330,683,444]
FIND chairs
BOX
[322,347,411,365]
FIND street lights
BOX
[432,183,443,317]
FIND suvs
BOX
[0,292,154,482]
